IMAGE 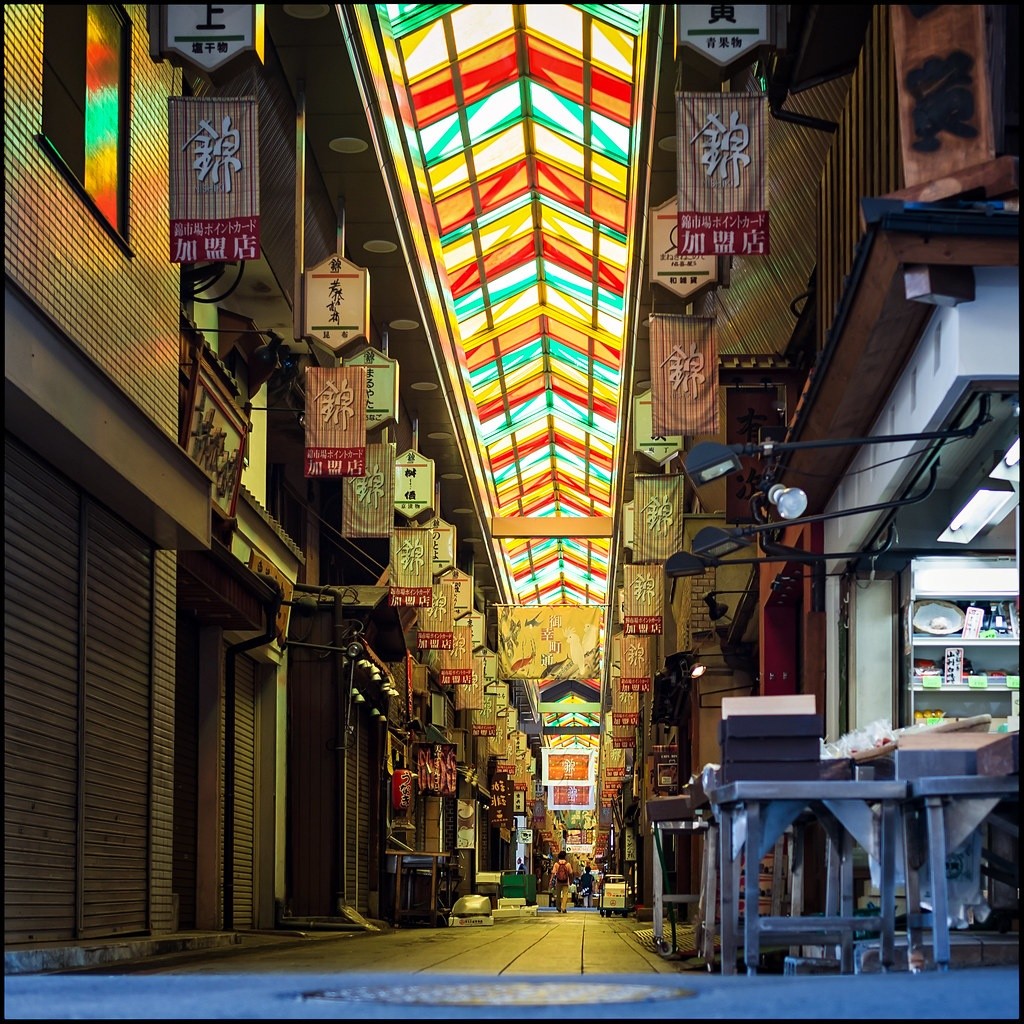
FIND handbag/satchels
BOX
[568,883,577,893]
[549,875,556,888]
[580,885,591,896]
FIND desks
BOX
[649,821,709,951]
[711,781,905,977]
[896,775,1021,978]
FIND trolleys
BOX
[597,874,632,919]
[499,870,539,906]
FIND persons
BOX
[550,851,574,913]
[517,857,527,874]
[580,867,595,907]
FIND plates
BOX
[914,600,966,636]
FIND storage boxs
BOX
[646,796,695,823]
[897,733,1019,782]
[716,694,826,785]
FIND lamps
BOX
[767,478,808,518]
[665,521,897,577]
[692,456,943,552]
[250,406,306,425]
[678,392,995,488]
[684,660,707,679]
[284,636,365,661]
[192,326,284,378]
[702,589,755,621]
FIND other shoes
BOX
[556,907,561,912]
[562,909,566,913]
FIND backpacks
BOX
[557,861,569,883]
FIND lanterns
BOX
[393,768,412,811]
